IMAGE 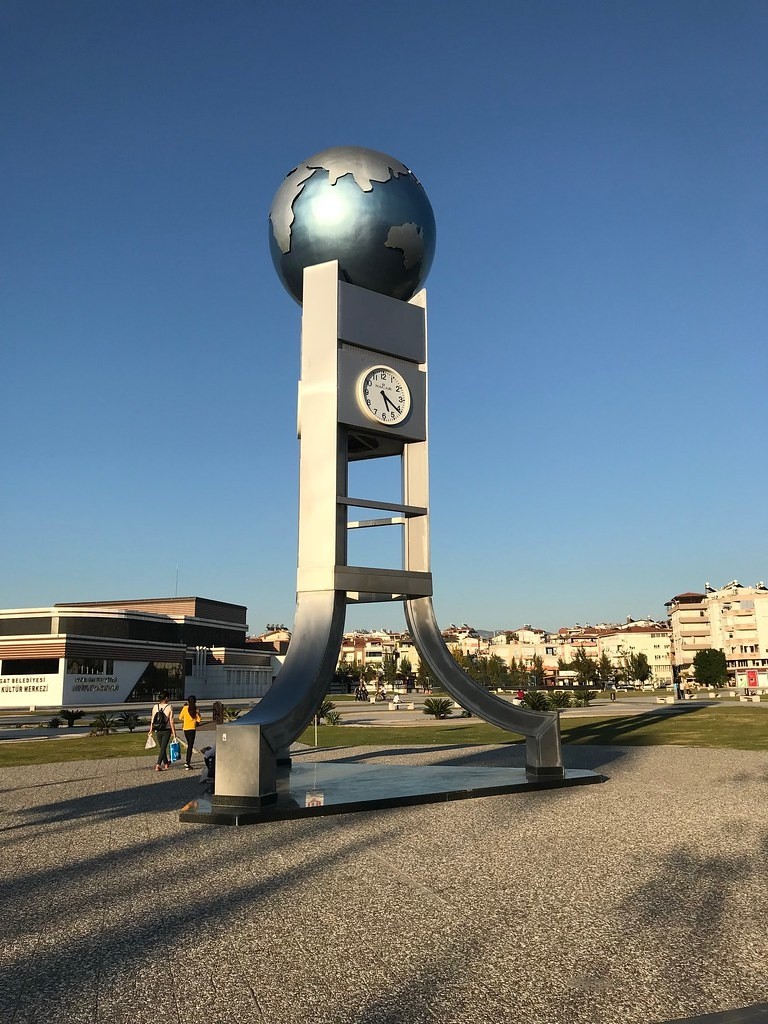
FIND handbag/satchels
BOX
[144,735,157,750]
[169,738,181,764]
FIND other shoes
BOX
[164,760,171,769]
[186,765,193,771]
[156,766,162,771]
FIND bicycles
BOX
[376,693,392,702]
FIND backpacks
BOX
[152,703,170,730]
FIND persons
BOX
[204,741,216,778]
[147,692,176,771]
[514,689,525,700]
[179,695,201,770]
[394,693,401,709]
[674,680,691,699]
[354,686,368,702]
[379,685,386,701]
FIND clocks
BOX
[356,364,411,425]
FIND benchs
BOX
[389,701,414,710]
[739,695,760,703]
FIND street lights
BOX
[425,676,430,685]
[531,676,536,686]
